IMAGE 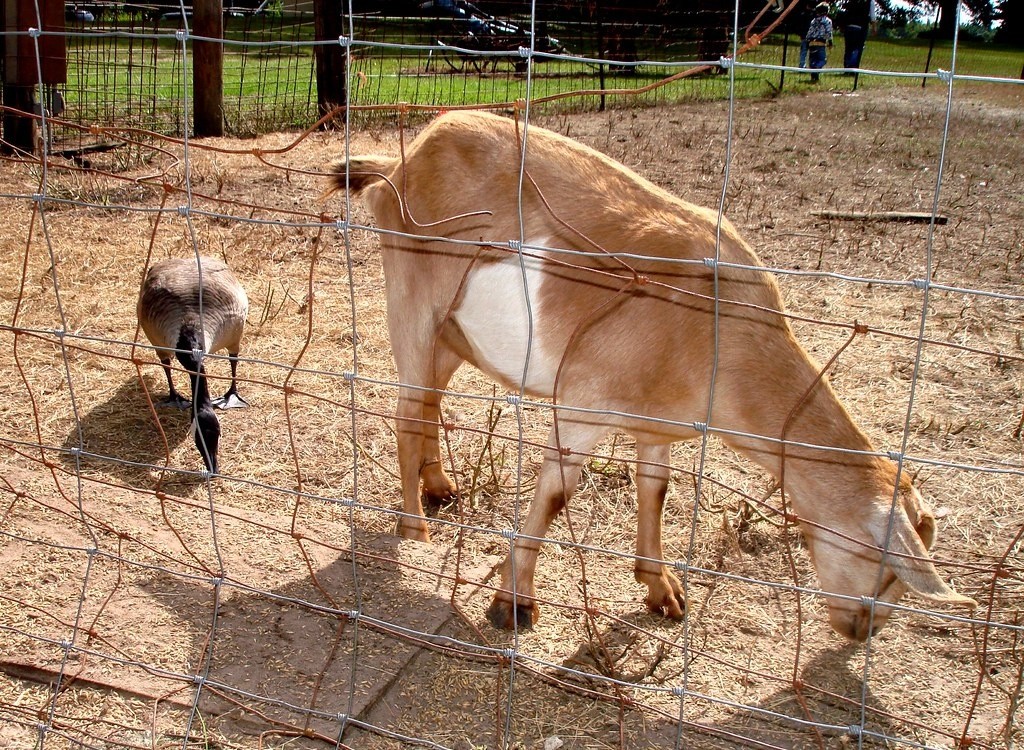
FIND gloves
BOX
[827,40,832,47]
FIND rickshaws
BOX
[419,0,583,73]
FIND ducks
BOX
[136,253,248,474]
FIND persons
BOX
[797,2,817,73]
[838,0,868,76]
[806,2,833,80]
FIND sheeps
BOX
[328,111,981,643]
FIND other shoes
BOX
[844,72,855,76]
[811,72,819,81]
